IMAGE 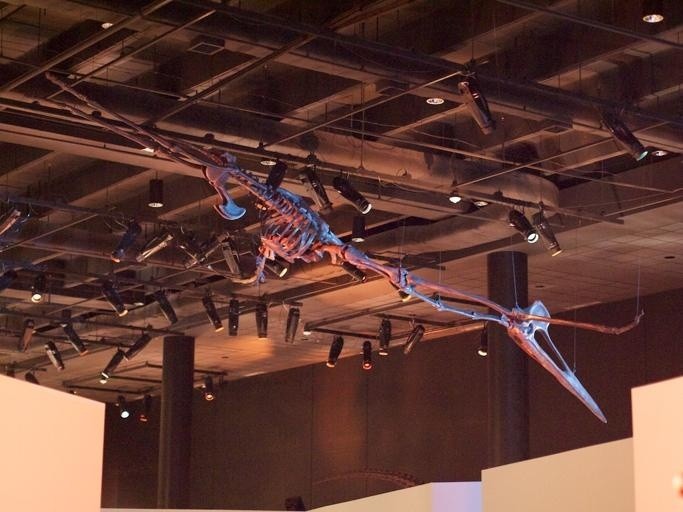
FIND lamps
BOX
[125,333,152,361]
[177,232,207,265]
[135,228,174,264]
[327,176,371,213]
[600,106,650,161]
[352,211,365,246]
[327,337,344,367]
[248,249,287,279]
[256,302,269,340]
[342,261,367,281]
[377,318,391,355]
[457,67,496,135]
[44,343,63,370]
[103,350,124,379]
[112,222,141,265]
[284,307,299,342]
[156,293,177,325]
[478,329,488,357]
[201,295,224,332]
[63,325,87,357]
[529,211,562,259]
[146,178,163,207]
[507,210,540,244]
[404,324,425,354]
[228,299,240,337]
[102,285,128,317]
[299,168,333,217]
[255,163,287,209]
[361,340,372,370]
[16,316,37,354]
[117,395,130,417]
[203,377,215,400]
